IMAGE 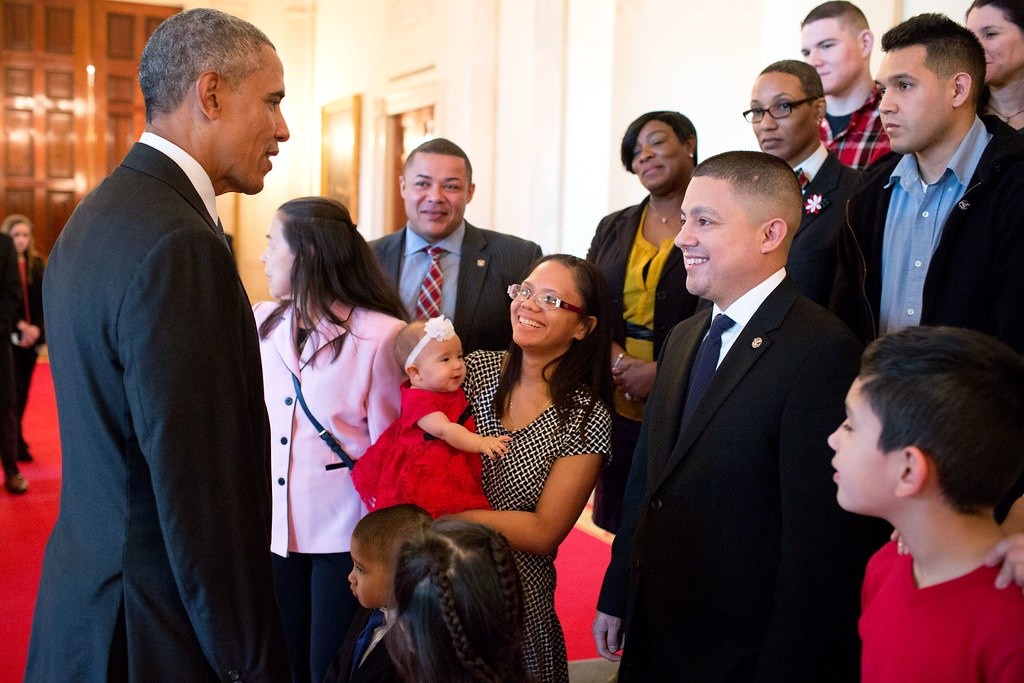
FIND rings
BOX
[625,392,631,401]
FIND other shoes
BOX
[16,447,32,461]
[4,473,29,493]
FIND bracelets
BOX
[614,352,627,368]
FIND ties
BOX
[793,168,809,190]
[415,246,447,320]
[217,217,233,256]
[676,313,737,451]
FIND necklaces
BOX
[649,201,679,224]
[989,104,1024,123]
[510,397,552,431]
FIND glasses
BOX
[506,284,590,317]
[742,94,825,123]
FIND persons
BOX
[251,196,412,683]
[827,326,1024,683]
[24,7,289,683]
[743,60,862,308]
[350,314,513,521]
[455,254,616,683]
[966,0,1024,137]
[382,516,539,683]
[0,214,46,494]
[323,504,434,683]
[802,1,892,170]
[827,13,1024,359]
[587,111,699,535]
[592,152,866,683]
[365,138,542,360]
